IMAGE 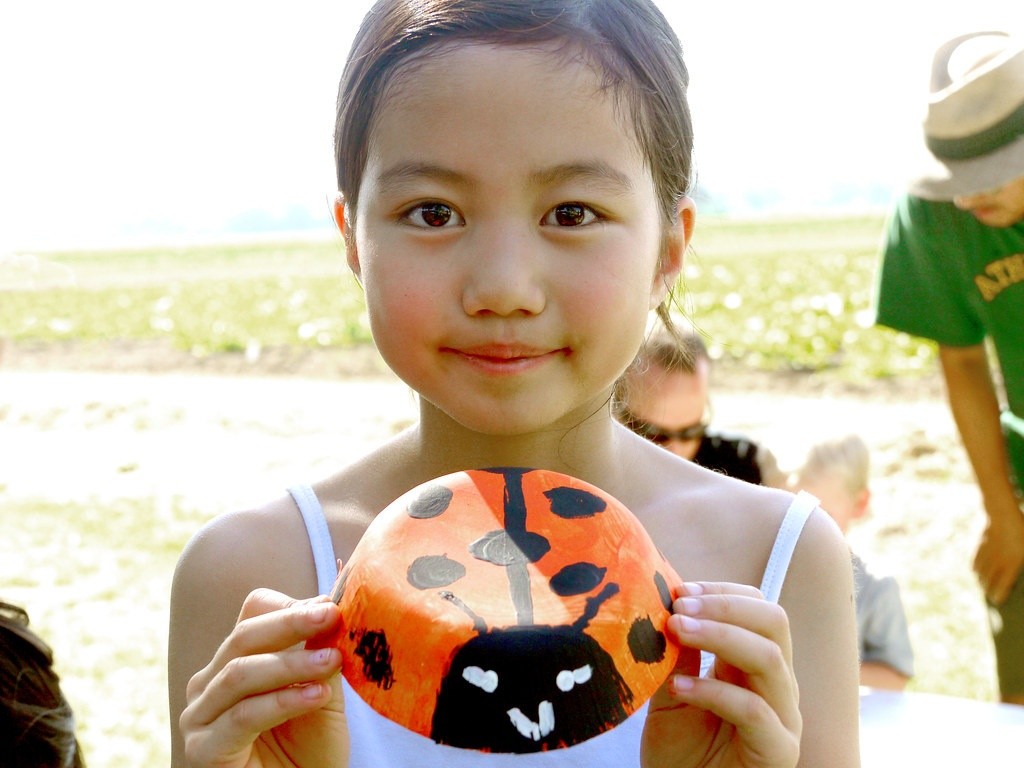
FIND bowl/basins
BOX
[322,468,682,754]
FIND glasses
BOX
[614,394,713,448]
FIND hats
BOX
[904,29,1024,200]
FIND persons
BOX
[784,426,917,692]
[168,0,862,768]
[876,31,1024,708]
[0,603,86,768]
[617,315,782,488]
